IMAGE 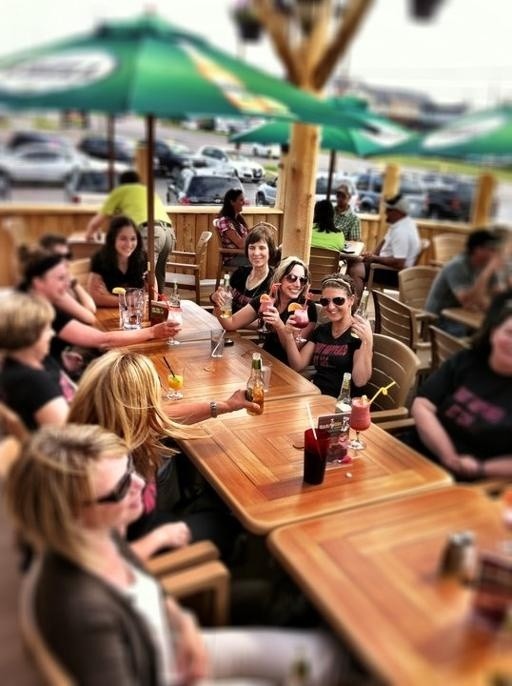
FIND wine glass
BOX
[165,307,183,345]
[292,303,310,343]
[257,302,273,333]
[350,395,370,451]
[165,366,184,401]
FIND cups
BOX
[258,358,273,393]
[209,328,227,358]
[303,427,331,486]
[117,287,146,329]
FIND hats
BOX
[384,195,410,213]
[337,183,352,197]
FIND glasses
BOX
[93,451,135,505]
[319,296,347,307]
[285,273,308,286]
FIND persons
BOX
[4,421,347,686]
[1,290,511,556]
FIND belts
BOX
[138,222,172,229]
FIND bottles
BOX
[334,371,352,439]
[245,352,266,416]
[170,279,181,311]
[438,530,475,580]
[221,272,234,318]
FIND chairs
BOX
[0,436,219,575]
[70,258,90,291]
[19,557,231,685]
[0,403,29,442]
[3,218,34,286]
